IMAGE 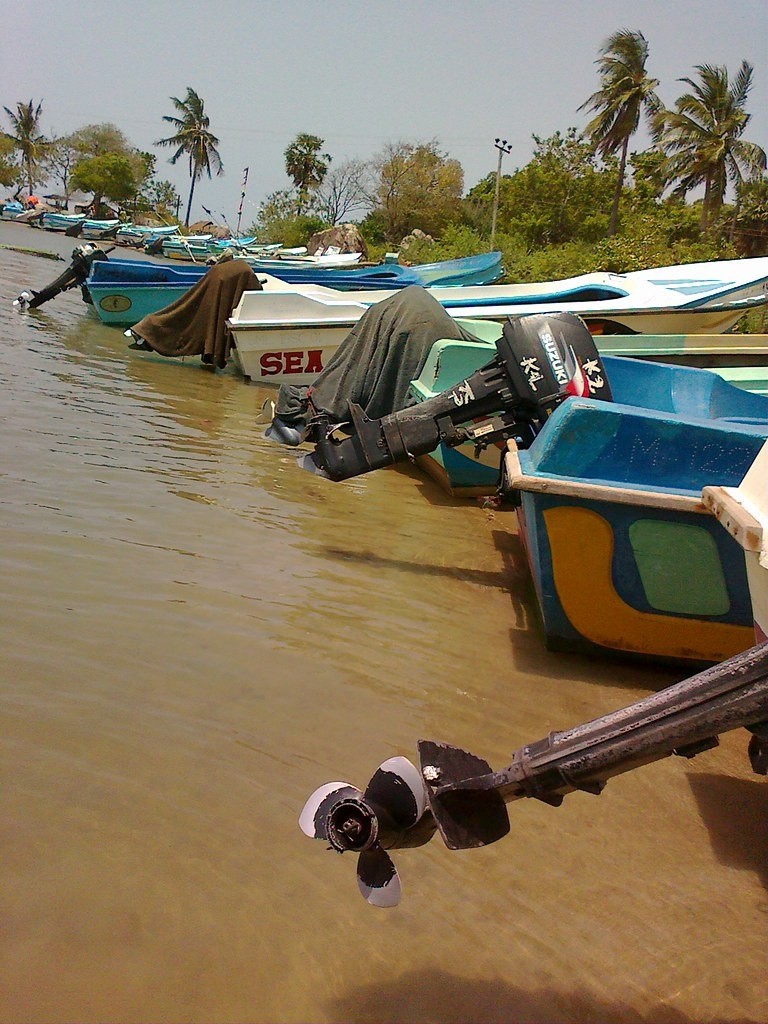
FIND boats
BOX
[0,198,768,908]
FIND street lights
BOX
[490,137,513,252]
